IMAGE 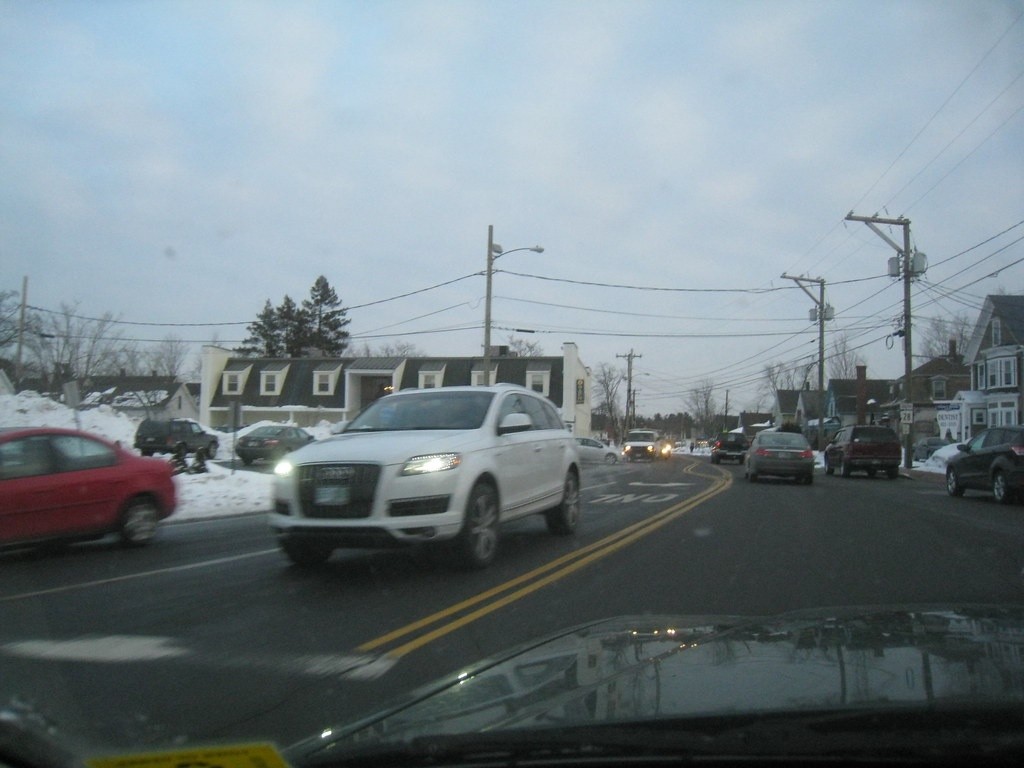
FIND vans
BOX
[623,430,661,462]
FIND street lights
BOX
[483,245,544,384]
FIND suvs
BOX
[134,417,220,460]
[266,382,584,568]
[709,432,749,466]
[824,424,901,479]
[945,425,1024,503]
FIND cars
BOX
[744,431,815,484]
[1,426,176,552]
[661,439,672,460]
[912,437,952,461]
[236,425,317,466]
[575,437,623,465]
